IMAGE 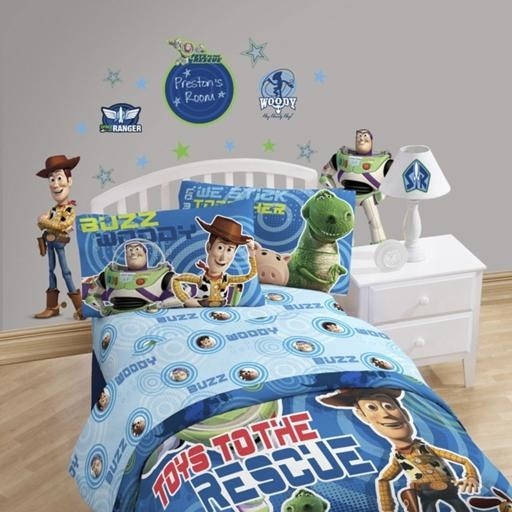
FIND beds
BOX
[68,157,512,512]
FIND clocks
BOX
[375,238,408,271]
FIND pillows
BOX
[177,180,356,298]
[75,199,266,318]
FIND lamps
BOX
[379,144,451,263]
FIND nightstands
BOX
[344,234,486,388]
